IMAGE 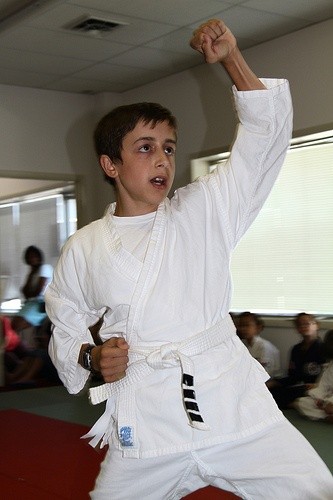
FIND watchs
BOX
[82,344,97,376]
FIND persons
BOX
[44,19,333,500]
[229,313,333,423]
[0,247,102,385]
